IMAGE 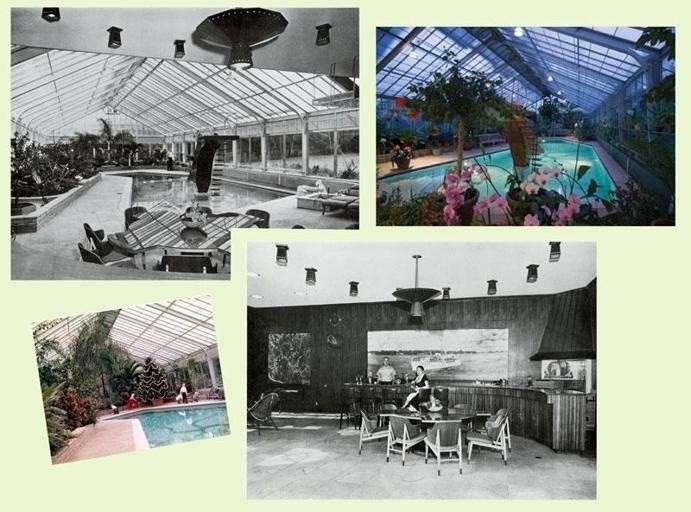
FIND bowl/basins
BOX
[179,212,207,229]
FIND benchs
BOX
[476,132,508,149]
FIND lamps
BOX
[513,26,565,98]
[271,241,568,320]
[37,4,336,73]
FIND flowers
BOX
[389,144,416,169]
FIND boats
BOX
[545,361,575,380]
[412,351,463,375]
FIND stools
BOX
[319,184,359,220]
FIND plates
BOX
[428,408,441,412]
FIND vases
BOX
[397,158,412,169]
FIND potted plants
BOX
[10,129,54,218]
[625,133,676,227]
[502,100,570,227]
[462,136,475,151]
[402,42,520,226]
[136,355,171,408]
[431,143,442,156]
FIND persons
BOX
[166,148,173,171]
[376,357,396,425]
[180,383,188,404]
[405,366,429,411]
[129,393,137,408]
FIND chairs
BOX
[334,382,518,477]
[73,197,272,277]
[247,390,281,437]
